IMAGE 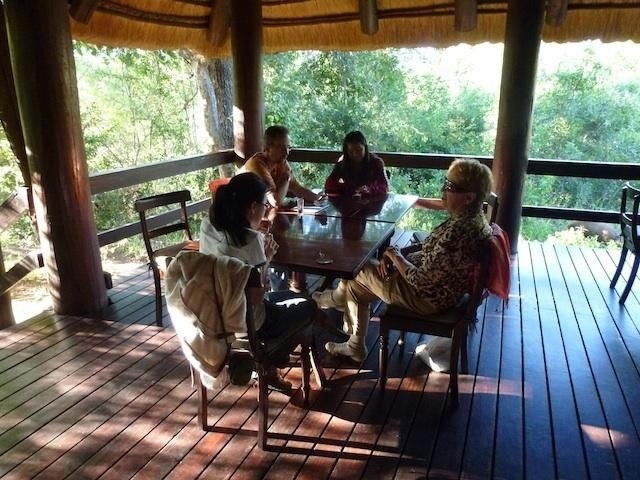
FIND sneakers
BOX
[310,287,348,314]
[251,366,294,393]
[324,339,370,363]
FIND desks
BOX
[186,190,420,385]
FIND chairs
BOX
[208,176,233,206]
[609,181,640,306]
[177,250,316,451]
[410,191,499,248]
[377,169,395,261]
[377,223,501,405]
[133,190,201,327]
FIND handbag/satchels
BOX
[226,348,254,387]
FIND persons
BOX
[328,195,388,241]
[413,197,447,210]
[235,126,328,209]
[311,159,495,361]
[325,131,389,194]
[198,173,317,392]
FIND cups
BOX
[296,198,304,214]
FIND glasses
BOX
[442,176,474,195]
[256,201,271,210]
